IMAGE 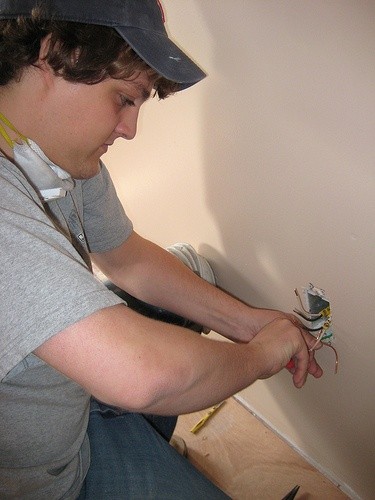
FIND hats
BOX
[0,1,207,93]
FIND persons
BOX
[0,1,327,500]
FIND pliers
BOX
[278,484,300,500]
